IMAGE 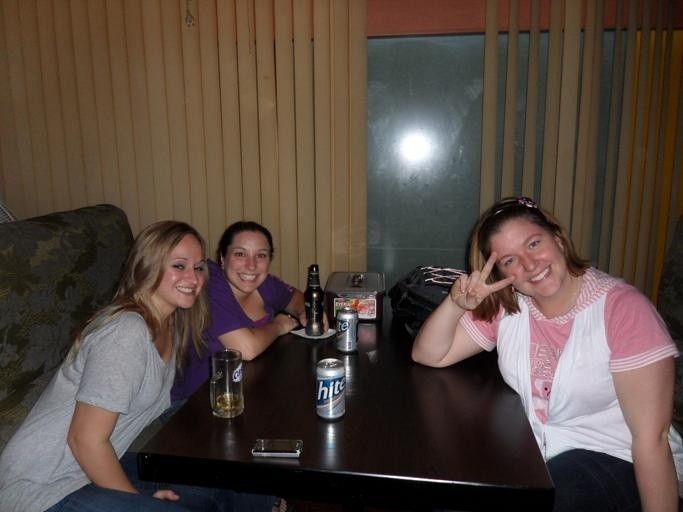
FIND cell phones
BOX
[252,438,304,458]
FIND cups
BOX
[206,350,245,418]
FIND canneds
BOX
[335,306,360,353]
[314,357,346,420]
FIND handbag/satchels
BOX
[388,264,467,320]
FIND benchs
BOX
[3,202,189,450]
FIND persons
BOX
[169,221,331,407]
[0,219,288,512]
[410,195,683,512]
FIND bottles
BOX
[304,263,325,337]
